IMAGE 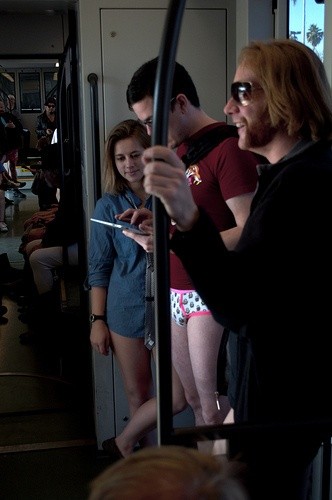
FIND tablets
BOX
[90,217,146,236]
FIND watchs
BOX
[89,314,105,322]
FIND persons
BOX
[125,53,268,456]
[0,95,85,345]
[87,119,188,457]
[144,36,332,500]
[88,445,249,500]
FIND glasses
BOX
[138,96,175,127]
[48,105,55,108]
[230,80,264,106]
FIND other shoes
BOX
[102,437,123,461]
[13,190,27,198]
[12,182,26,188]
[0,222,8,232]
